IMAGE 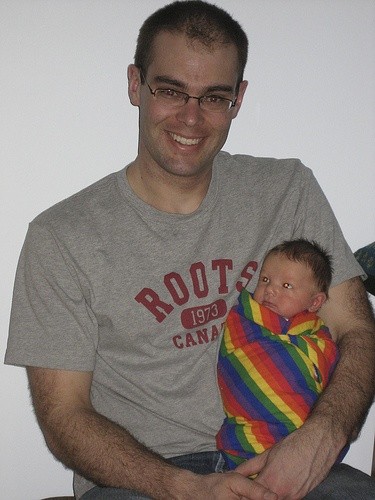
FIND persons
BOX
[215,237,339,471]
[4,0,375,500]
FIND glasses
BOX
[136,63,240,113]
